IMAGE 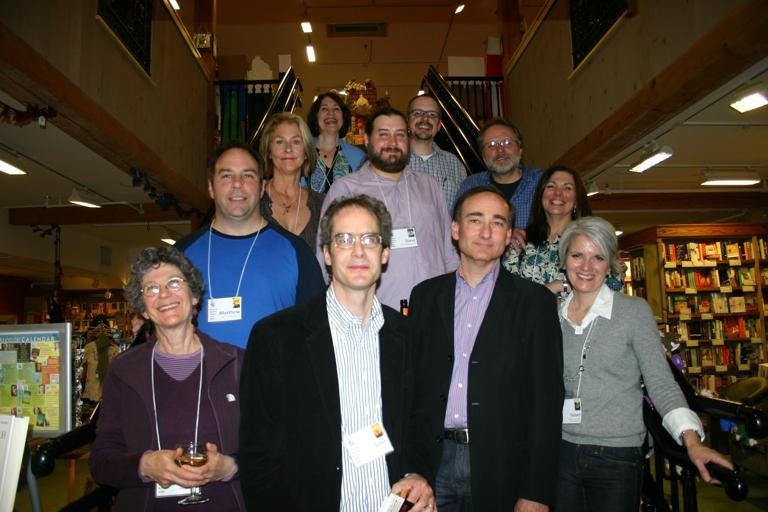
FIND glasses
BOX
[408,110,439,118]
[331,232,381,249]
[140,276,189,297]
[483,138,518,148]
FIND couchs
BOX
[709,378,767,451]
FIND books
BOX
[664,238,768,392]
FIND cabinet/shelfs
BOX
[618,222,768,394]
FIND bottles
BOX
[125,320,129,334]
[113,319,119,329]
[400,299,407,315]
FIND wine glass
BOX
[176,441,211,504]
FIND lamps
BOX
[0,142,113,209]
[23,297,138,371]
[583,181,599,197]
[729,76,767,115]
[628,140,674,174]
[697,164,762,188]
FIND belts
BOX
[444,428,469,445]
